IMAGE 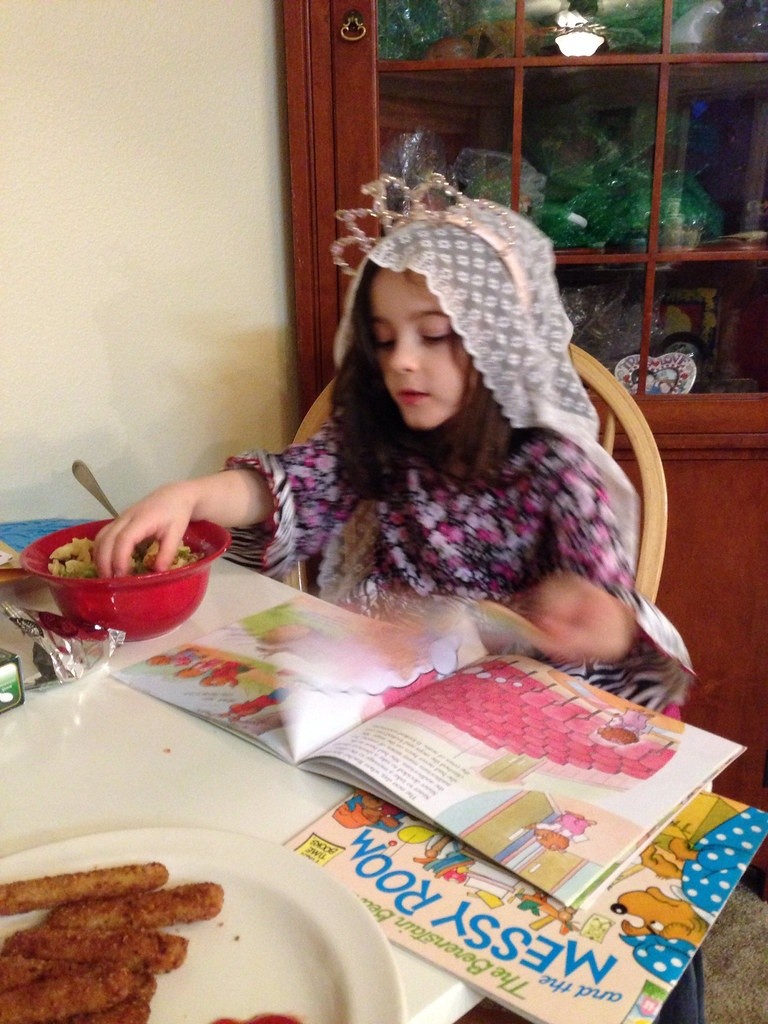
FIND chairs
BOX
[292,347,669,672]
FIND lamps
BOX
[556,1,605,58]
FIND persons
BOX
[92,199,636,657]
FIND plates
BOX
[0,826,408,1024]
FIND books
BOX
[284,789,768,1024]
[112,594,748,906]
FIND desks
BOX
[0,557,742,1024]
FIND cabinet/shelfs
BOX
[280,0,768,874]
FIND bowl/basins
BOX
[21,515,231,643]
